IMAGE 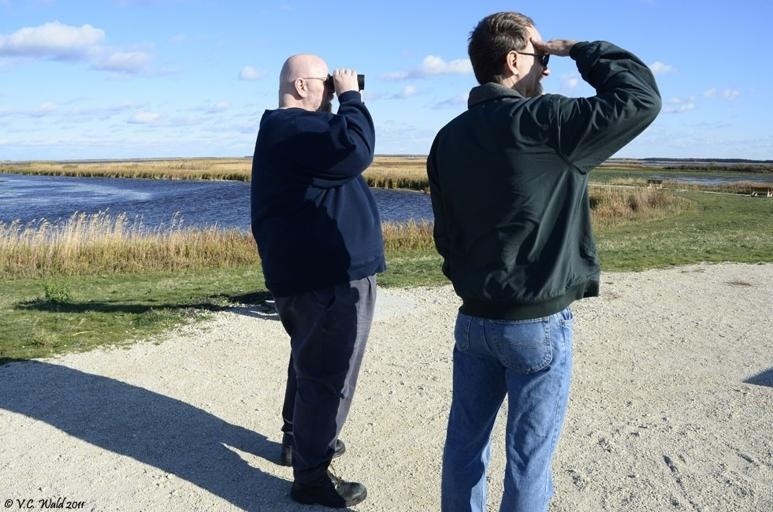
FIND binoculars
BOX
[329,74,365,91]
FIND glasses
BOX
[303,73,330,87]
[518,52,550,66]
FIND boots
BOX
[291,470,367,507]
[282,434,345,466]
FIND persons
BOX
[426,11,662,512]
[250,55,388,508]
